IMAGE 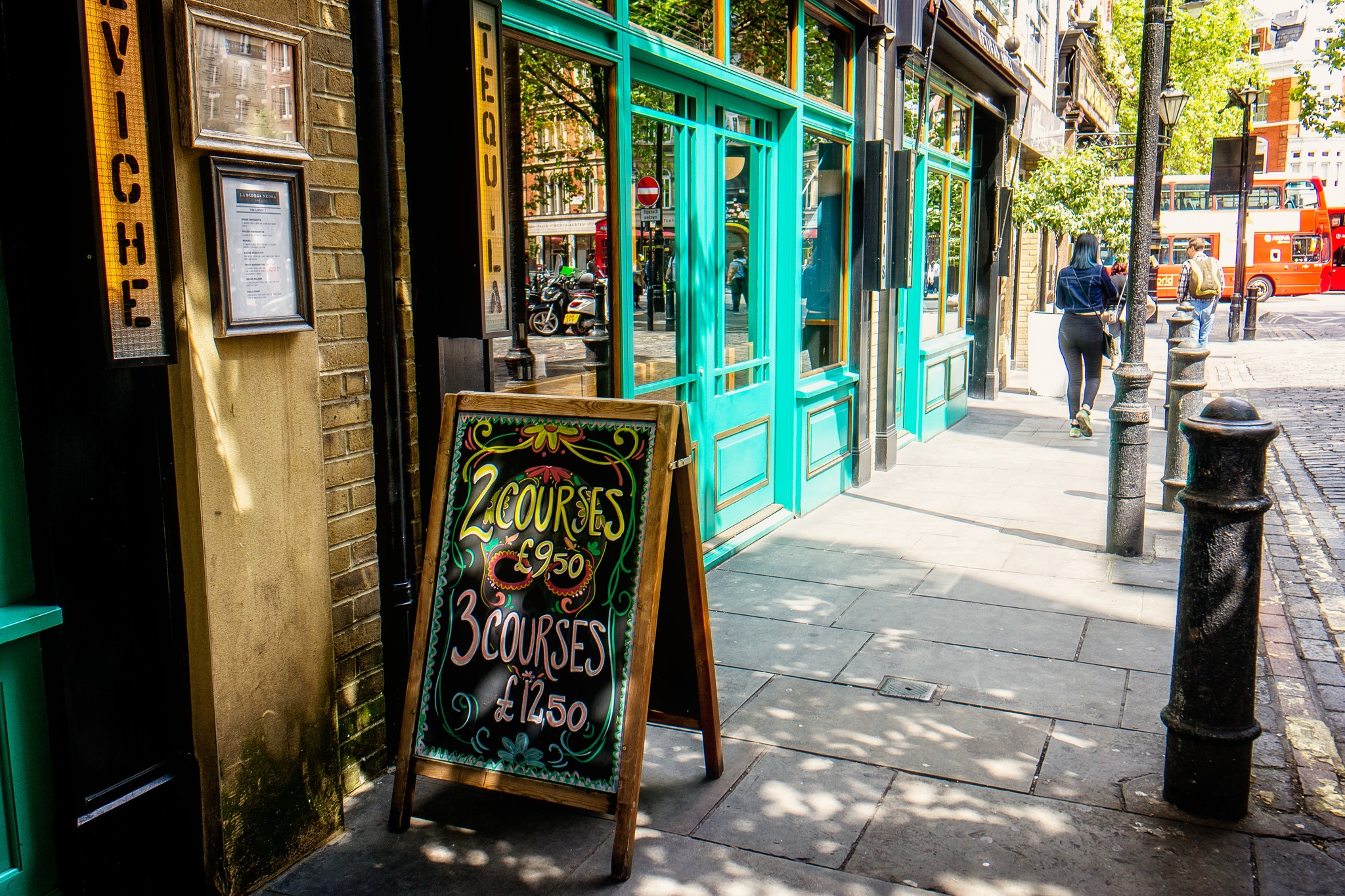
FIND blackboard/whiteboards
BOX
[398,388,719,809]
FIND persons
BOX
[1107,258,1128,367]
[585,247,829,368]
[1055,233,1118,438]
[1177,236,1226,348]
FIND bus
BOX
[1094,172,1345,302]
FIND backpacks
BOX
[1187,258,1220,299]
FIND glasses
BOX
[1187,245,1194,251]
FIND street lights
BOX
[1218,76,1263,343]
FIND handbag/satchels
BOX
[1102,330,1113,361]
[1102,310,1119,324]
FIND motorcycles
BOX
[524,267,610,338]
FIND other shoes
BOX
[1110,363,1117,369]
[1076,410,1092,437]
[1069,428,1083,437]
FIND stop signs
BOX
[636,176,661,207]
